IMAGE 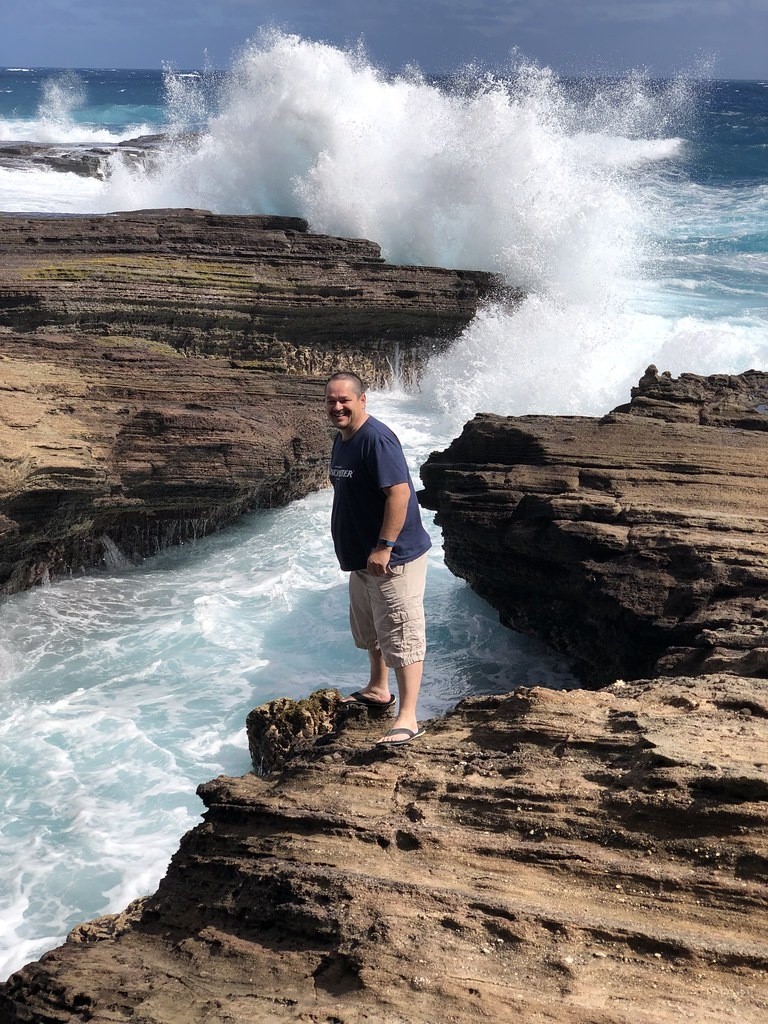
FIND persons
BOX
[324,370,430,746]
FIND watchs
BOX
[376,539,397,549]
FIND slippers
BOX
[376,728,426,747]
[339,692,397,706]
